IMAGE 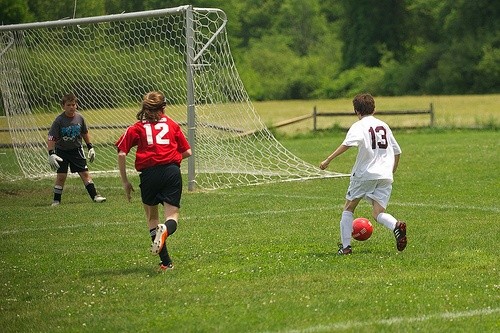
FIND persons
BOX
[115,91,192,271]
[320,94,407,254]
[47,94,106,206]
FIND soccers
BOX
[352,218,372,240]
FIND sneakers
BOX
[337,243,352,255]
[157,261,174,271]
[150,223,168,256]
[94,195,106,202]
[52,200,59,206]
[394,221,407,251]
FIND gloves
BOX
[49,150,63,172]
[87,143,95,163]
